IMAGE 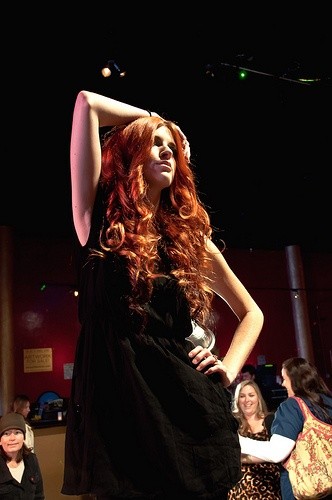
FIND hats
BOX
[0,413,26,440]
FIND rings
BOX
[211,354,218,365]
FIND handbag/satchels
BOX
[281,396,332,500]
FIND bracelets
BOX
[147,109,152,117]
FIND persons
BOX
[60,90,264,500]
[229,365,283,500]
[238,357,332,500]
[0,413,45,500]
[11,395,35,454]
